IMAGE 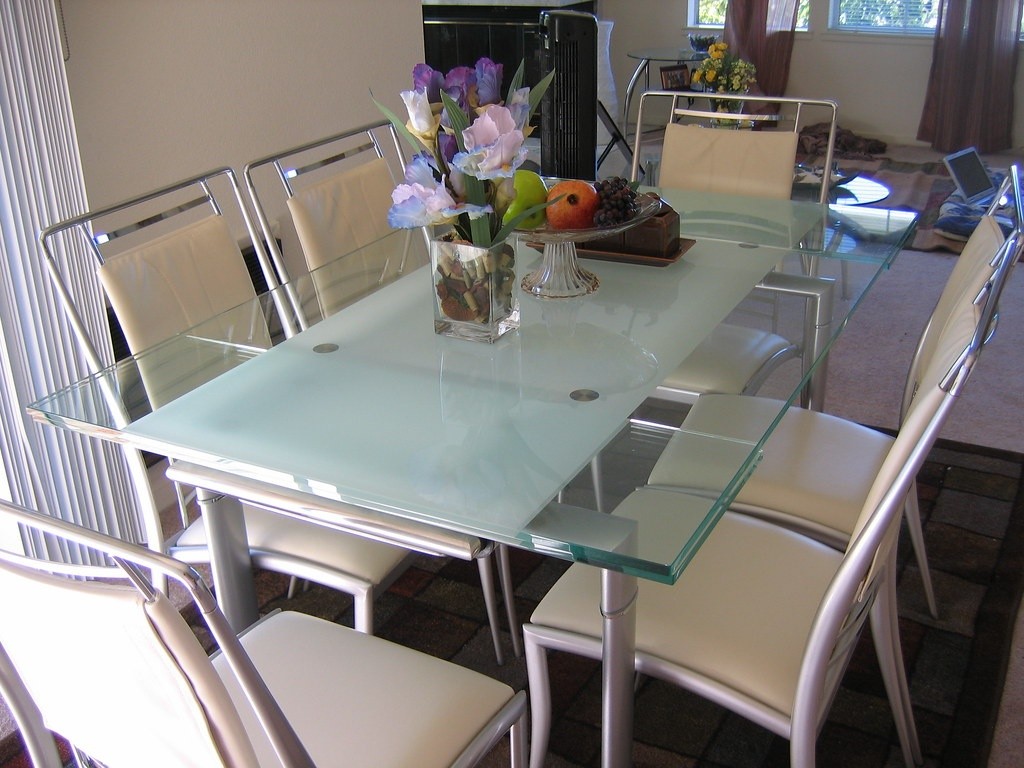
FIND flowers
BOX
[692,43,757,127]
[367,52,568,263]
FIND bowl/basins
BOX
[688,33,719,54]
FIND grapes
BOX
[592,177,637,227]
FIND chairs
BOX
[643,160,1024,768]
[522,238,1019,768]
[588,89,839,531]
[0,503,528,768]
[41,166,416,638]
[242,120,435,335]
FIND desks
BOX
[617,45,712,139]
[21,178,920,768]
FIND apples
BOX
[501,169,549,229]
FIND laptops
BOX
[942,146,1024,219]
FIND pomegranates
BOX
[546,181,599,228]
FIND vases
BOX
[706,97,744,132]
[425,228,522,341]
